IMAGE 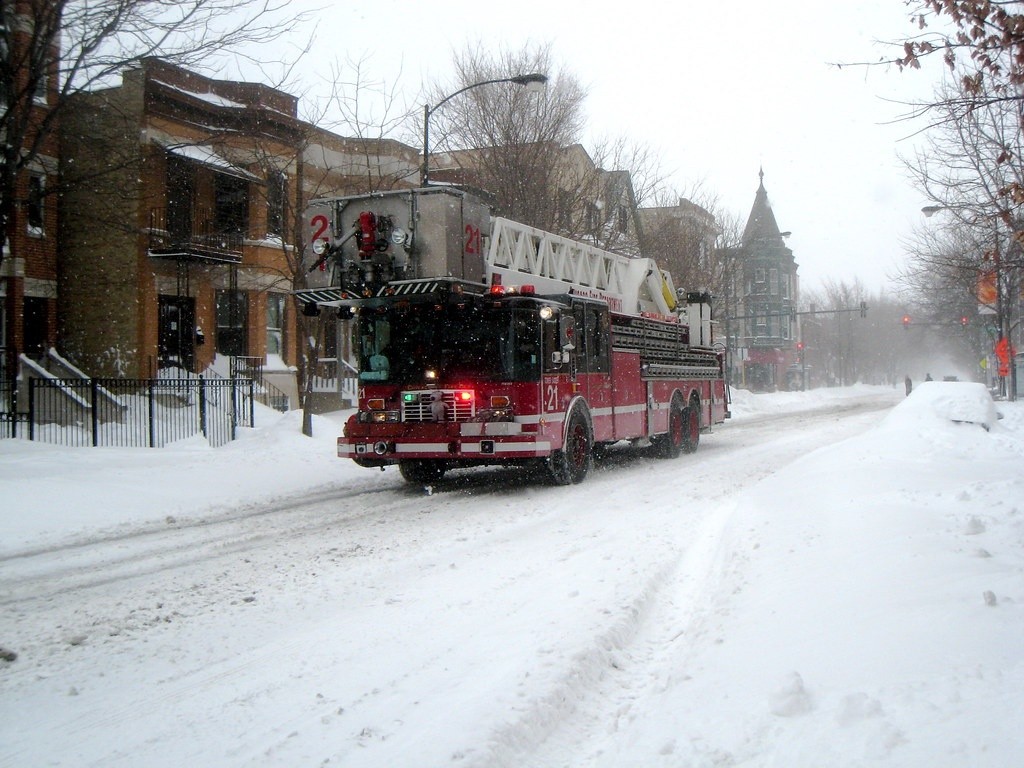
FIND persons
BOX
[905,375,912,396]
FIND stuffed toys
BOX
[428,391,450,422]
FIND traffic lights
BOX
[961,313,968,325]
[903,314,910,329]
[797,343,802,355]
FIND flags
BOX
[978,268,999,315]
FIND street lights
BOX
[421,74,549,195]
[723,230,791,384]
[921,204,1008,397]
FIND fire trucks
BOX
[297,185,733,486]
